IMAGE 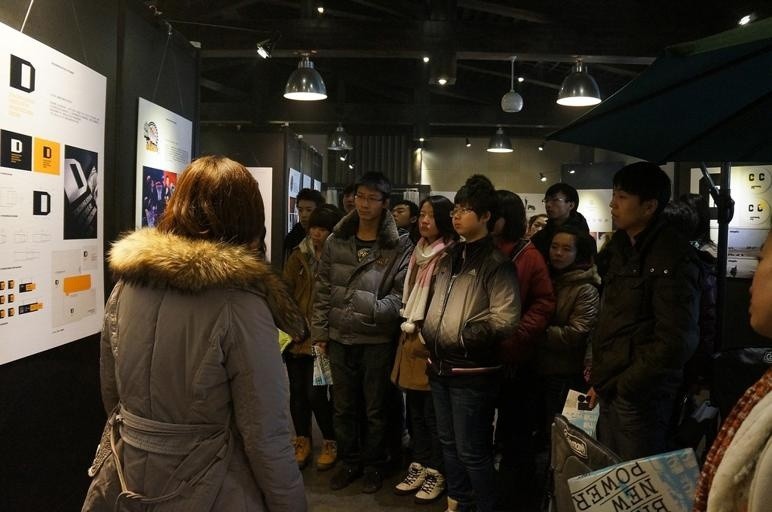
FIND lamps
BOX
[501,56,525,112]
[282,54,329,101]
[326,123,354,152]
[487,126,514,152]
[555,64,603,107]
[339,152,348,162]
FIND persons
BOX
[696,228,772,512]
[139,167,179,231]
[81,154,314,511]
[265,161,725,510]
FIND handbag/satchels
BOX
[311,345,333,387]
[548,414,627,512]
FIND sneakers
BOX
[316,439,338,470]
[295,437,313,476]
[360,466,383,493]
[393,462,427,495]
[413,467,446,505]
[329,464,361,491]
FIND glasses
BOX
[450,207,473,217]
[542,198,570,205]
[354,194,383,204]
